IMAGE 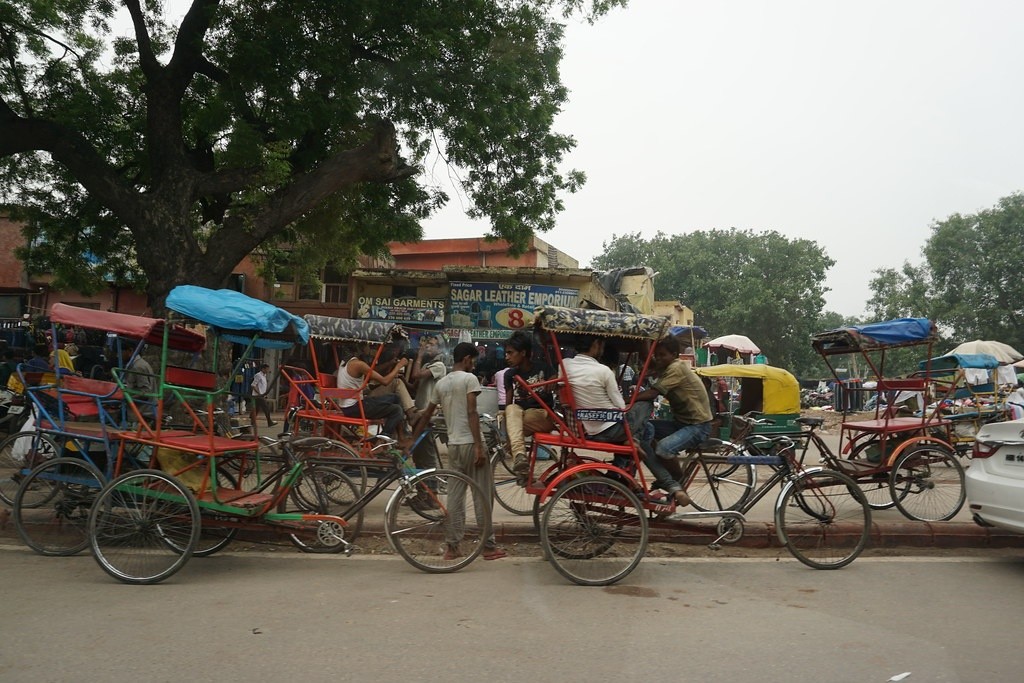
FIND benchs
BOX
[839,377,1008,438]
[21,359,400,519]
[531,381,647,457]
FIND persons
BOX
[495,362,510,411]
[631,334,718,507]
[0,348,18,391]
[336,342,429,449]
[557,333,656,481]
[277,361,316,438]
[702,378,719,419]
[409,342,509,561]
[401,334,447,511]
[503,327,558,487]
[47,349,75,376]
[618,359,636,406]
[25,345,49,372]
[249,364,278,427]
[113,338,159,471]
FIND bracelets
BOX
[415,360,422,364]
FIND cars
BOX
[964,417,1024,535]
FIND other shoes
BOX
[402,495,432,506]
[512,456,530,471]
[516,474,536,487]
[674,490,691,506]
[413,500,440,510]
[268,421,277,426]
[408,409,425,426]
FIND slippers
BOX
[483,549,508,560]
[445,552,462,560]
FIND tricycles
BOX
[0,284,1024,589]
